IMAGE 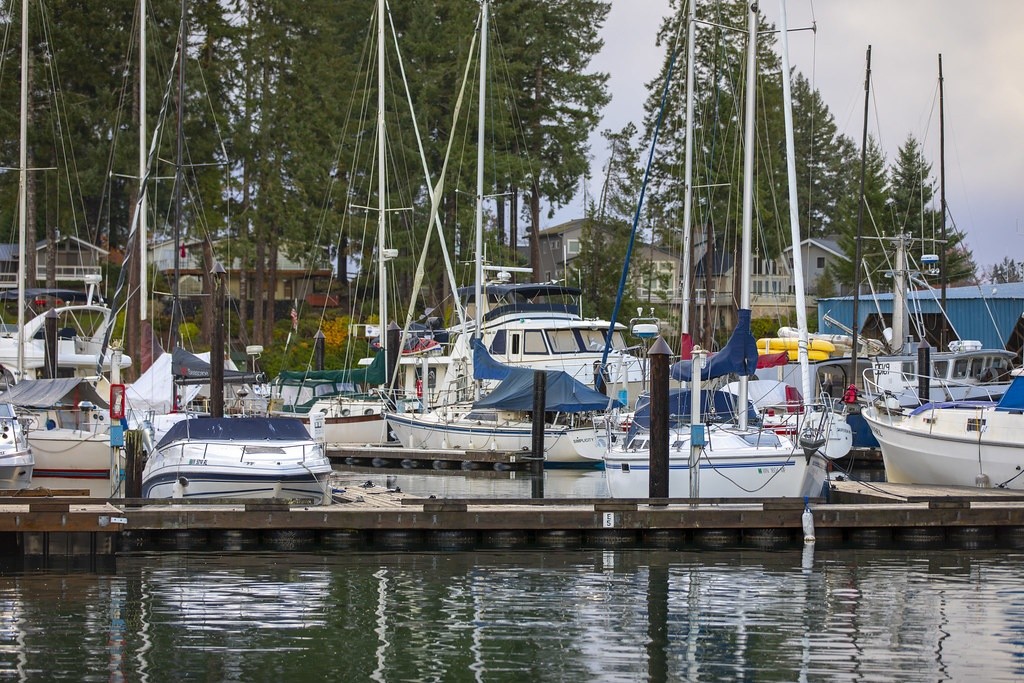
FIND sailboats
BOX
[1,1,1023,500]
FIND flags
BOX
[290,306,297,331]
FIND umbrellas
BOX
[180,242,185,268]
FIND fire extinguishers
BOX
[416,376,423,398]
